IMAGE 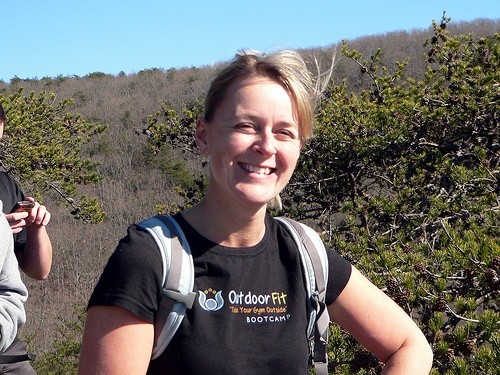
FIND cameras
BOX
[9,200,35,214]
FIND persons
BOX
[79,49,434,375]
[0,103,53,375]
[0,200,28,355]
